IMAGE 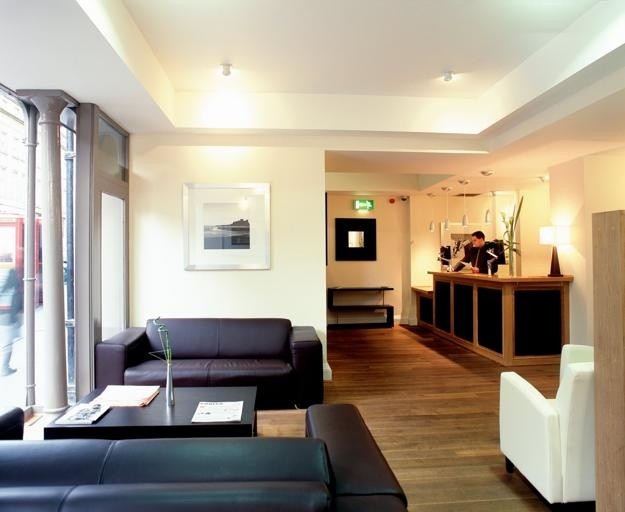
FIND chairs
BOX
[500,344,596,512]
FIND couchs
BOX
[95,317,324,411]
[0,405,410,512]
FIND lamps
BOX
[221,64,231,77]
[539,225,572,278]
[459,180,469,227]
[481,170,493,224]
[442,72,455,81]
[441,187,452,230]
[428,193,436,231]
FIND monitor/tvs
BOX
[488,241,504,266]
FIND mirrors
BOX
[334,217,377,261]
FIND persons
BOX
[0,252,23,376]
[446,231,499,275]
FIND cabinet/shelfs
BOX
[327,287,394,330]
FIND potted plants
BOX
[151,317,176,407]
[501,195,524,275]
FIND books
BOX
[91,383,161,408]
[190,400,244,422]
[53,403,111,424]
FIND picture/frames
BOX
[183,182,271,270]
